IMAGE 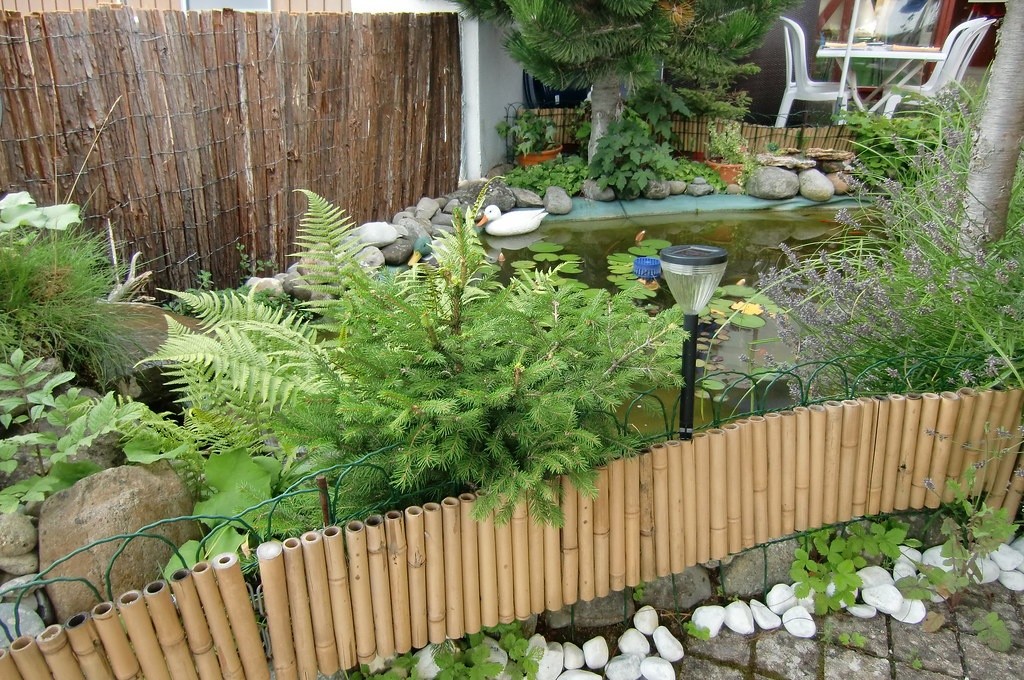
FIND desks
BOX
[815,42,946,118]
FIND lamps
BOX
[659,245,729,315]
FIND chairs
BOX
[878,16,997,119]
[775,16,852,127]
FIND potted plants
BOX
[495,111,563,167]
[703,120,751,184]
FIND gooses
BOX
[475,204,548,236]
